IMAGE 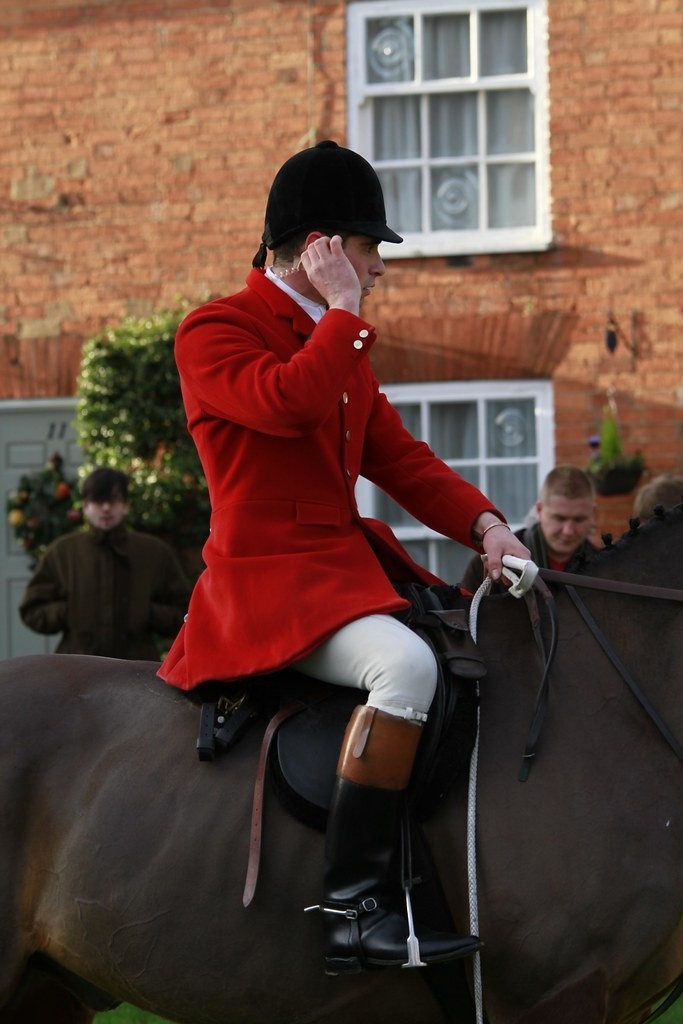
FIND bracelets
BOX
[481,522,511,545]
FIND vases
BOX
[591,466,645,499]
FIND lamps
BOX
[603,309,641,374]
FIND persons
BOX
[463,465,598,594]
[17,468,192,660]
[156,138,531,976]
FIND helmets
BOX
[253,140,402,266]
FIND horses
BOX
[0,466,683,1024]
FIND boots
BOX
[319,705,482,976]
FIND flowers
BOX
[588,402,643,469]
